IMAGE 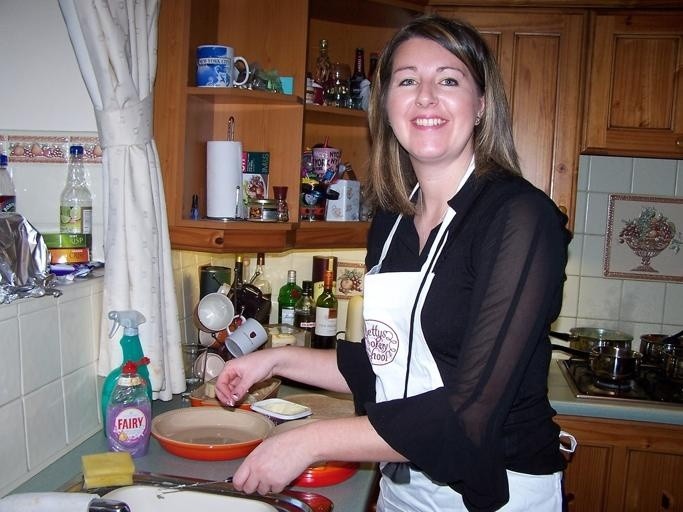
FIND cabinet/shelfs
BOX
[547,413,627,512]
[625,418,683,512]
[416,0,590,243]
[142,0,430,255]
[580,0,682,160]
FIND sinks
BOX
[55,469,311,512]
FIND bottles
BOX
[0,154,16,213]
[249,252,271,299]
[228,255,243,297]
[59,145,92,235]
[313,39,350,107]
[349,46,378,110]
[277,269,339,350]
[272,185,289,222]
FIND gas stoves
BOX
[558,358,681,408]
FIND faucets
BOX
[1,491,130,512]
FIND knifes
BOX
[158,476,232,490]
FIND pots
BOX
[550,327,633,353]
[551,344,643,384]
[639,330,683,358]
[657,343,683,380]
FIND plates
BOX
[151,406,276,460]
[269,418,360,488]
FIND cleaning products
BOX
[100,309,152,437]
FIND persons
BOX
[215,14,578,512]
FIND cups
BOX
[195,45,250,88]
[193,283,272,381]
[313,148,341,181]
